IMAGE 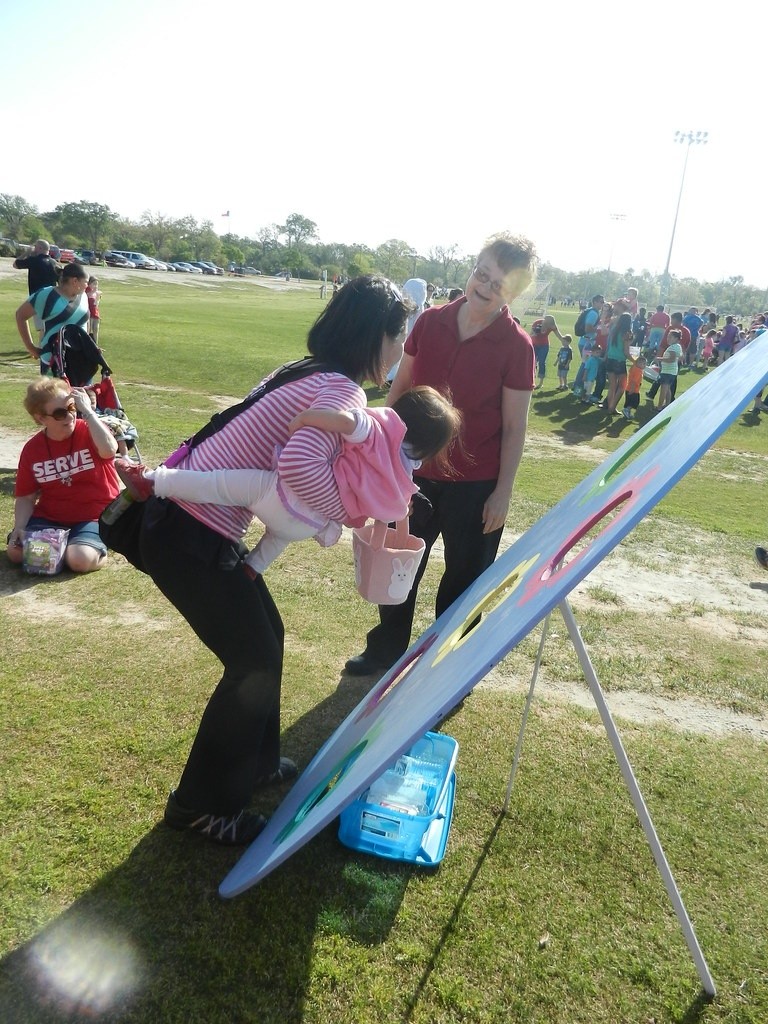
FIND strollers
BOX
[52,323,142,463]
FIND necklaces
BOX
[42,426,77,487]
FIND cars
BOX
[275,271,292,278]
[19,243,225,276]
[244,266,261,275]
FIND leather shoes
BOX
[344,650,398,675]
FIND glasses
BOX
[473,265,514,297]
[44,404,76,420]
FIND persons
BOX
[534,288,768,421]
[136,273,409,846]
[84,276,101,348]
[304,269,464,305]
[16,261,93,377]
[4,372,121,575]
[12,238,60,344]
[342,236,538,675]
[111,383,460,588]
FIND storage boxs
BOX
[336,732,458,865]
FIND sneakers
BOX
[260,755,300,787]
[556,384,569,392]
[163,790,268,842]
[621,408,635,419]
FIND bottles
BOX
[366,753,446,805]
